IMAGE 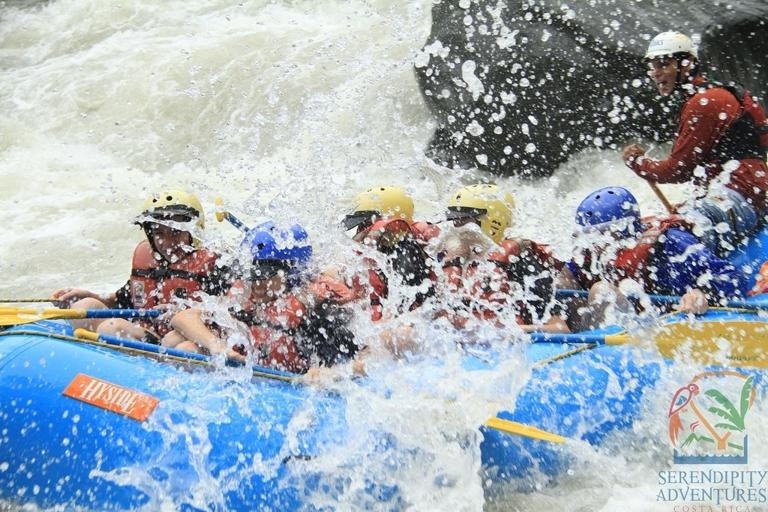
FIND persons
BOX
[53,187,767,389]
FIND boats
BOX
[0,223,768,511]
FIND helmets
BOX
[644,32,699,65]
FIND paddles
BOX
[526,320,768,368]
[74,328,567,444]
[0,307,168,325]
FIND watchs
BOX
[621,32,767,273]
